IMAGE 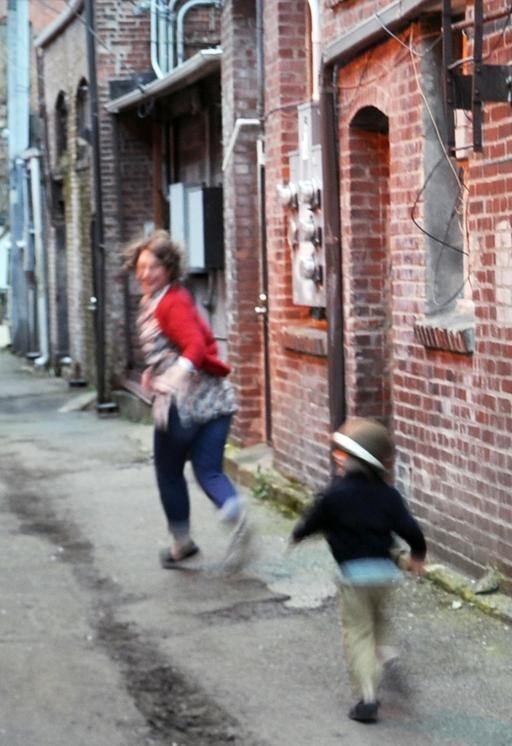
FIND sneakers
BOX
[349,699,382,722]
[158,541,200,566]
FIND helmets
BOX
[330,415,394,474]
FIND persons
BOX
[112,228,256,575]
[284,415,431,720]
